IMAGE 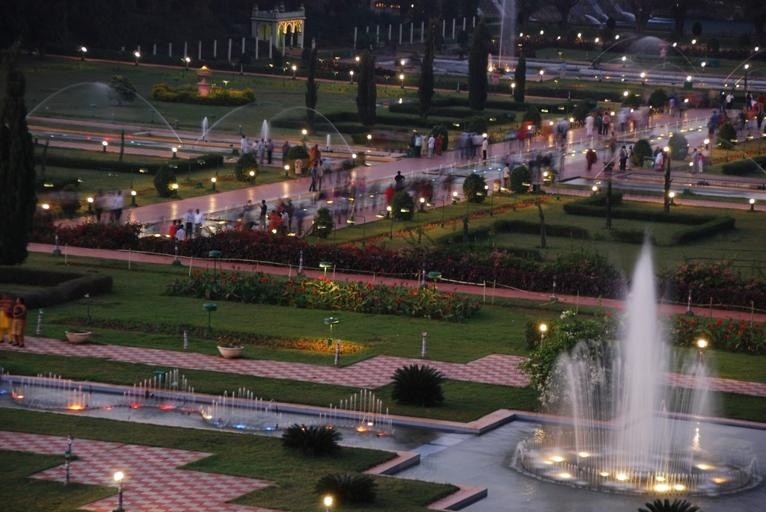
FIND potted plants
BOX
[217,335,246,358]
[64,328,92,344]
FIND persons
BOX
[241,137,273,164]
[170,209,203,242]
[96,189,124,222]
[691,149,704,174]
[616,107,640,132]
[268,200,304,237]
[394,171,405,184]
[411,130,448,158]
[627,145,633,159]
[597,112,610,135]
[620,145,627,170]
[652,146,664,172]
[708,111,728,138]
[502,163,510,187]
[737,105,763,135]
[311,144,321,165]
[508,118,570,149]
[0,293,26,347]
[585,116,594,136]
